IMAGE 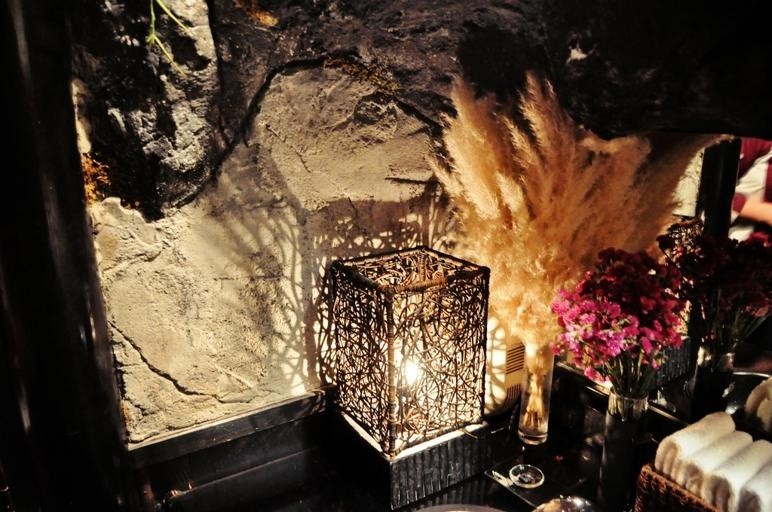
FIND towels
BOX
[654,411,772,512]
[743,372,772,444]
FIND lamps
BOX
[325,246,491,512]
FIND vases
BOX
[598,385,648,512]
[691,345,735,423]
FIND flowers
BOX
[668,218,771,354]
[553,249,690,402]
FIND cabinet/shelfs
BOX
[485,354,689,512]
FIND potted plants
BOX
[423,66,683,447]
